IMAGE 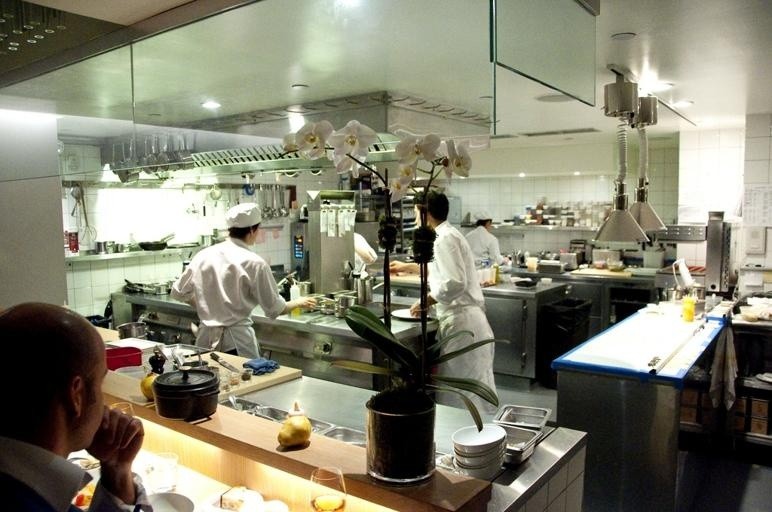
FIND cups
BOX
[511,254,521,268]
[682,301,695,323]
[221,368,255,393]
[525,256,540,274]
[201,234,215,247]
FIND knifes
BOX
[210,353,241,373]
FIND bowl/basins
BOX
[541,277,553,286]
[515,281,537,287]
[453,423,507,483]
[685,284,706,300]
[116,320,150,340]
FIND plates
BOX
[147,493,195,512]
[71,458,102,476]
[755,373,772,383]
[391,308,430,320]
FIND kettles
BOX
[350,273,384,306]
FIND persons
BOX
[465,210,509,270]
[389,191,500,414]
[353,232,378,279]
[0,302,154,511]
[170,202,317,360]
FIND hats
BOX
[226,202,262,229]
[473,209,493,220]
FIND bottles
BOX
[514,200,612,227]
[149,351,165,373]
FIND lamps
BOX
[593,63,668,244]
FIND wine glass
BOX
[306,467,350,512]
[105,400,136,418]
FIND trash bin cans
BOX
[537,297,592,390]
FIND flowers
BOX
[274,118,513,432]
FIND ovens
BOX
[606,283,662,330]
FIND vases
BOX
[365,393,440,488]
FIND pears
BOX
[278,415,311,447]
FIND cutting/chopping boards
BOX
[168,350,303,402]
[390,270,421,283]
[570,264,633,279]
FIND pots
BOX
[94,239,123,254]
[138,234,176,250]
[154,366,220,421]
[538,251,560,261]
[311,289,358,318]
